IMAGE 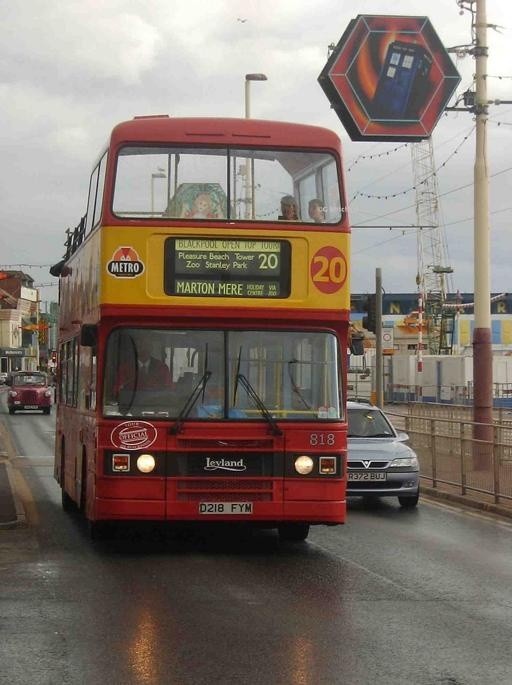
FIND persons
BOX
[111,335,176,401]
[278,196,301,221]
[307,199,328,224]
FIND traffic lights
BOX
[361,294,377,336]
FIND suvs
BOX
[343,395,422,509]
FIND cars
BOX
[0,368,59,415]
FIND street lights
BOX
[244,71,268,221]
[150,172,168,218]
[51,348,57,404]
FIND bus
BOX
[47,112,356,546]
